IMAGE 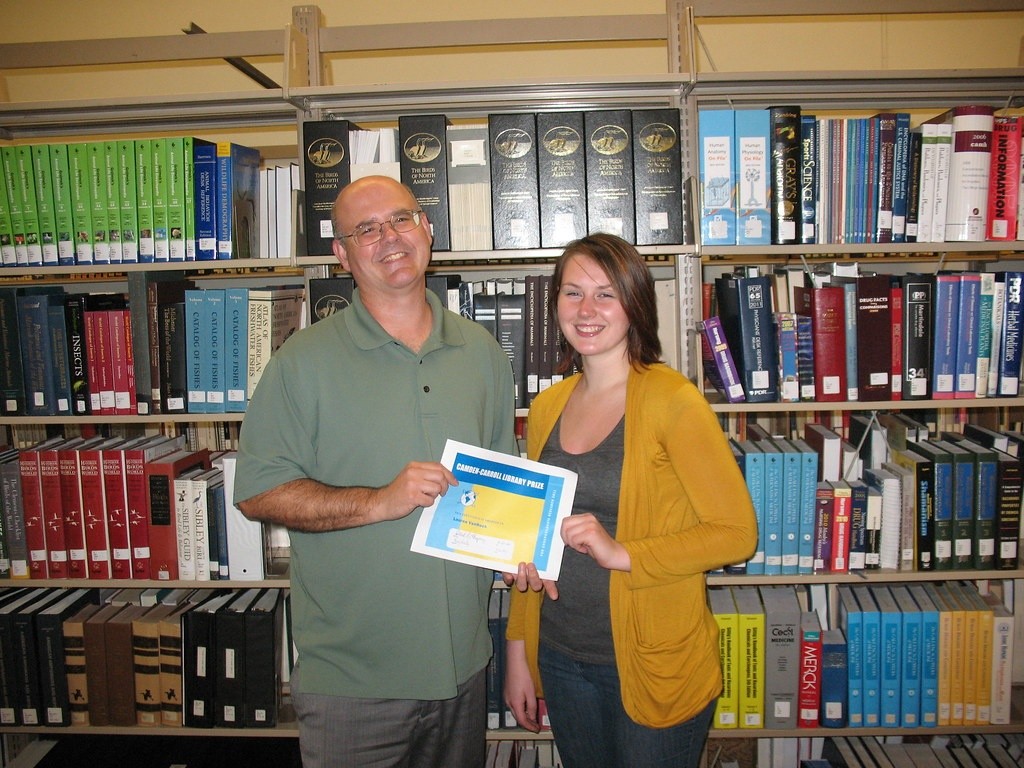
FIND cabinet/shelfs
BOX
[0,0,1024,768]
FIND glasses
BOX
[336,209,424,247]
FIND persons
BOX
[503,232,759,768]
[233,176,558,768]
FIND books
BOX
[0,271,308,417]
[701,261,1024,402]
[0,421,291,579]
[486,591,565,768]
[0,588,299,768]
[698,104,1024,245]
[495,417,530,582]
[721,734,1024,768]
[303,109,684,256]
[707,578,1024,728]
[0,137,301,267]
[709,406,1024,574]
[308,274,679,408]
